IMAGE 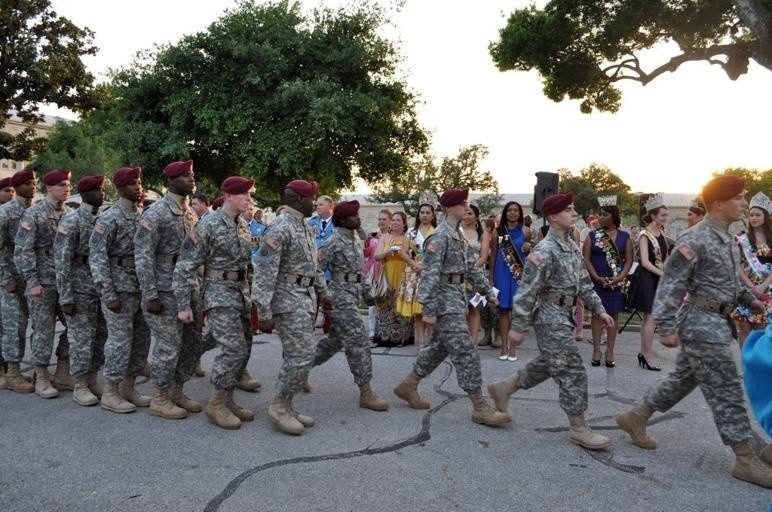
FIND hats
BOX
[538,190,575,218]
[286,180,320,196]
[437,187,470,207]
[0,176,13,190]
[77,174,106,195]
[112,165,142,189]
[703,175,745,206]
[11,169,36,188]
[219,175,257,195]
[333,200,361,218]
[43,168,72,187]
[163,160,194,179]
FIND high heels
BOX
[638,352,643,366]
[641,354,661,372]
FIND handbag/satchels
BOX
[360,266,390,305]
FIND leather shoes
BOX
[370,341,380,349]
[604,351,615,368]
[479,337,493,346]
[591,349,603,367]
[509,345,517,362]
[499,345,509,360]
[576,330,584,341]
[491,338,502,349]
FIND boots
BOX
[33,364,60,399]
[487,376,519,423]
[470,389,512,425]
[564,412,612,450]
[170,383,203,414]
[53,359,76,391]
[267,394,304,435]
[358,382,390,411]
[285,394,315,427]
[88,372,105,397]
[236,371,262,391]
[224,390,255,422]
[4,361,36,393]
[614,403,657,450]
[72,375,99,406]
[138,362,150,378]
[203,389,242,430]
[194,360,206,376]
[392,368,431,410]
[732,441,772,489]
[0,367,33,389]
[101,375,136,414]
[302,373,313,391]
[149,385,187,420]
[118,375,152,408]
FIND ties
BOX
[320,220,327,236]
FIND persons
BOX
[0,162,772,488]
